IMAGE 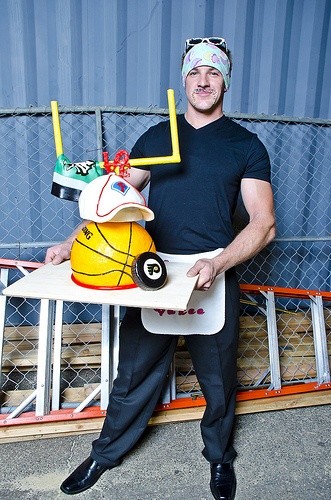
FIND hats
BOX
[79,172,154,223]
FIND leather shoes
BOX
[60,456,112,495]
[210,461,237,500]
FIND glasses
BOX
[185,37,227,57]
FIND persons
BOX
[43,38,275,500]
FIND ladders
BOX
[1,259,331,426]
